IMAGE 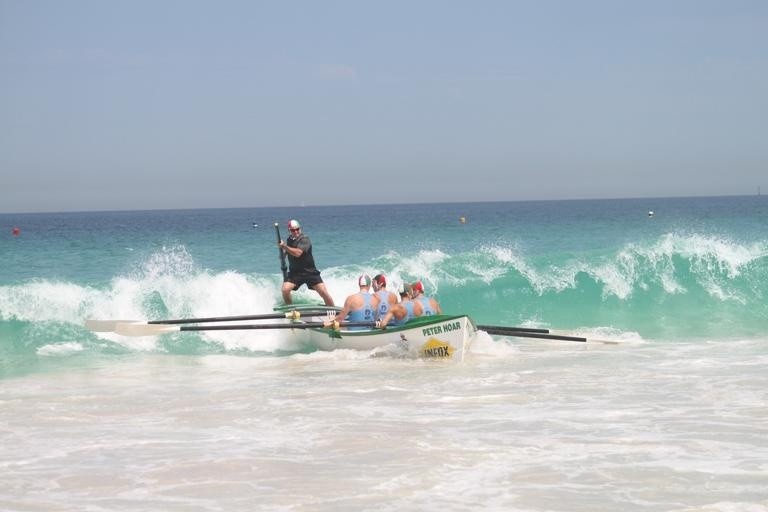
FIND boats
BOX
[272,275,478,361]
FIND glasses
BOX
[290,228,299,232]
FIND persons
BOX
[279,219,334,306]
[324,273,441,330]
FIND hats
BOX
[412,281,424,293]
[288,220,299,229]
[399,283,412,294]
[375,274,385,283]
[359,274,371,286]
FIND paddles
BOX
[114,319,381,345]
[488,331,624,345]
[84,311,341,332]
[476,325,572,334]
